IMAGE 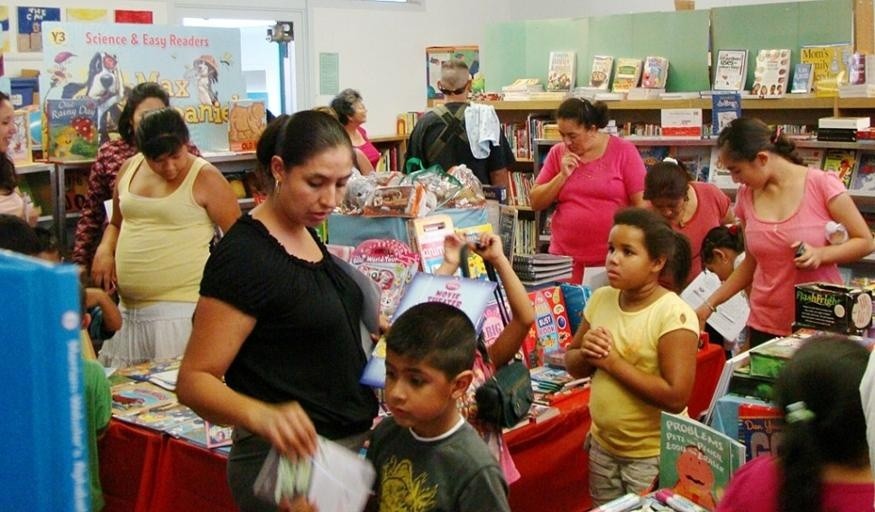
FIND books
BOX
[504,79,544,101]
[590,55,615,89]
[749,333,803,375]
[658,407,745,512]
[791,144,826,169]
[818,115,874,132]
[499,114,532,162]
[109,361,246,456]
[726,372,782,408]
[362,272,496,390]
[514,253,573,287]
[641,56,670,89]
[397,110,428,137]
[822,149,860,191]
[599,118,663,137]
[7,92,35,112]
[738,404,792,461]
[4,75,41,93]
[513,216,536,252]
[848,151,873,194]
[525,365,593,426]
[503,168,535,208]
[767,122,798,135]
[628,86,667,100]
[816,126,857,145]
[610,57,642,93]
[575,86,625,103]
[659,93,699,101]
[514,290,574,369]
[546,53,576,92]
[713,90,743,135]
[501,420,530,436]
[455,223,505,288]
[252,428,376,511]
[404,212,463,279]
[534,118,562,140]
[351,242,417,323]
[6,112,27,164]
[43,95,100,165]
[228,97,268,151]
[750,47,790,97]
[793,44,875,95]
[713,49,751,92]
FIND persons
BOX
[701,223,757,310]
[694,116,875,350]
[401,59,518,206]
[639,158,736,294]
[89,110,242,381]
[715,336,875,511]
[330,89,382,176]
[7,220,122,342]
[0,214,110,510]
[312,107,377,179]
[175,111,388,511]
[363,301,511,512]
[75,82,206,301]
[564,208,700,508]
[0,89,28,222]
[528,97,647,287]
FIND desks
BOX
[96,342,722,512]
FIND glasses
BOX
[439,81,469,95]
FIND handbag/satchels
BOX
[459,242,542,430]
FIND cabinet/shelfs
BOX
[45,155,263,250]
[12,158,59,256]
[367,135,407,171]
[480,96,875,256]
